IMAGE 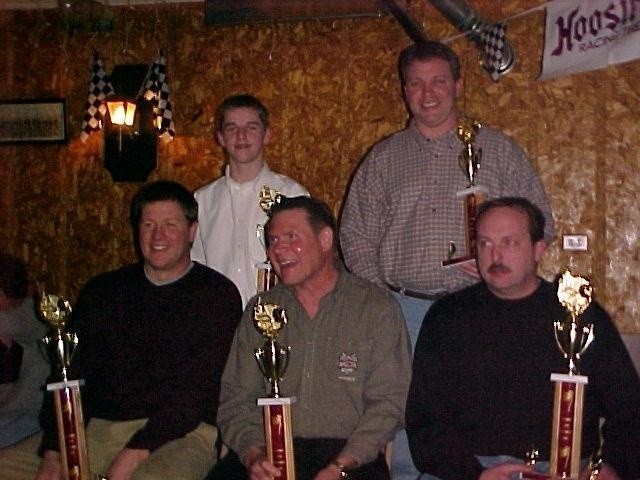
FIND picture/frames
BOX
[0,101,68,144]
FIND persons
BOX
[398,195,640,480]
[205,195,414,480]
[188,93,313,316]
[334,41,556,479]
[0,179,246,480]
[0,254,56,447]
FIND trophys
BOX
[35,289,82,374]
[256,184,286,264]
[249,297,293,397]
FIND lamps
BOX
[99,94,143,159]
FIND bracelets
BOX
[330,460,353,478]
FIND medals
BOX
[553,265,596,374]
[455,115,484,187]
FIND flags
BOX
[139,50,176,143]
[474,20,509,83]
[80,52,114,147]
